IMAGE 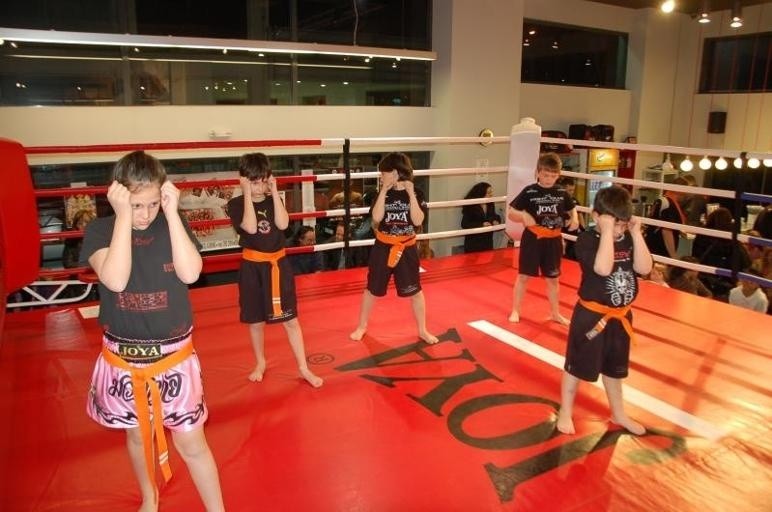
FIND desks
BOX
[676,222,755,258]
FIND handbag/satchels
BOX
[540,130,568,153]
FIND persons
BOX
[650,176,771,313]
[508,152,579,325]
[325,223,349,270]
[351,151,439,345]
[557,187,654,436]
[63,210,95,278]
[77,149,227,512]
[286,226,321,273]
[461,183,501,252]
[222,152,324,389]
[560,177,586,256]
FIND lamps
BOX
[691,0,714,24]
[730,0,744,28]
[680,153,693,171]
[1,0,438,62]
[715,157,728,170]
[699,155,712,169]
[662,153,674,172]
